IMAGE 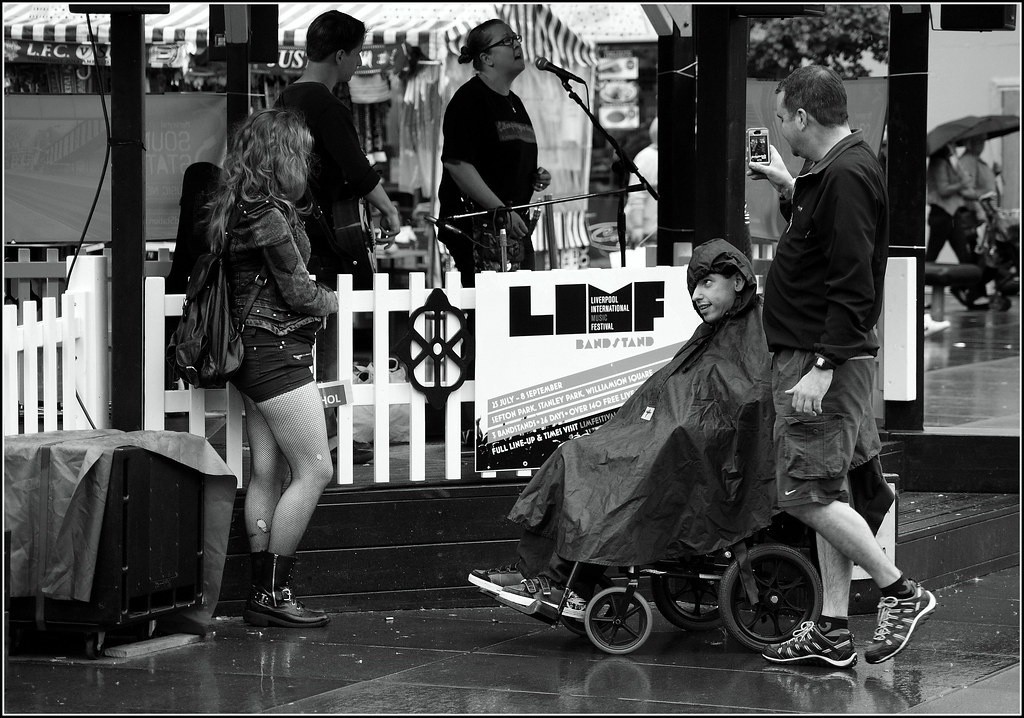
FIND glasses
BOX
[483,34,522,52]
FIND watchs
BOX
[814,357,836,371]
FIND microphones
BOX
[425,215,464,235]
[535,57,586,83]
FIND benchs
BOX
[926,264,982,321]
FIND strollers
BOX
[978,190,1020,314]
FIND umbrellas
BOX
[951,115,1020,186]
[926,116,992,177]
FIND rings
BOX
[539,184,543,187]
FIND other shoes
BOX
[923,313,950,337]
[970,293,991,314]
[950,285,968,308]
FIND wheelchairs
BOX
[478,508,825,656]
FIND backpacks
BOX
[167,205,291,390]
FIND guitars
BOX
[330,197,379,284]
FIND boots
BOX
[243,548,330,629]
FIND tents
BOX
[3,1,597,264]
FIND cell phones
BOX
[747,128,771,166]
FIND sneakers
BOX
[864,579,938,665]
[468,559,526,594]
[762,620,857,669]
[499,574,588,618]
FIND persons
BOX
[956,134,1005,307]
[436,19,552,454]
[624,116,658,249]
[195,105,339,627]
[746,64,937,669]
[271,9,400,465]
[925,137,990,311]
[468,237,777,618]
